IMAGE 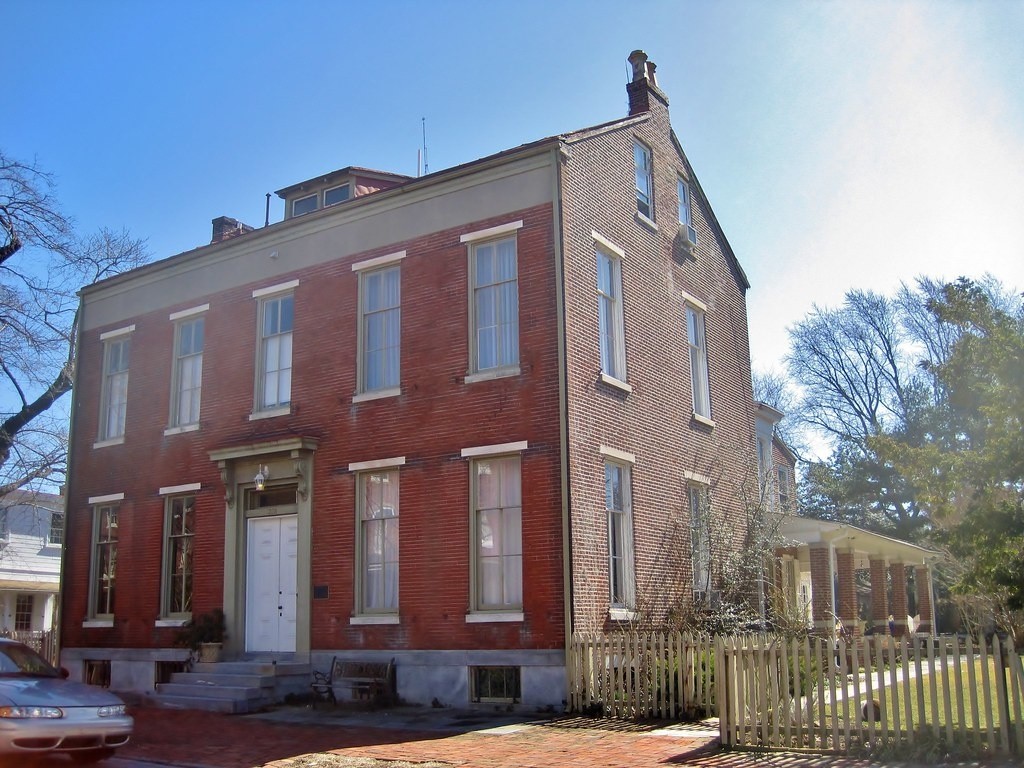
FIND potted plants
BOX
[170,608,230,664]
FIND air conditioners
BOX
[679,224,697,249]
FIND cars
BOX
[0,637,135,768]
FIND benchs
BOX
[307,656,398,717]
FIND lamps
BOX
[253,463,270,492]
